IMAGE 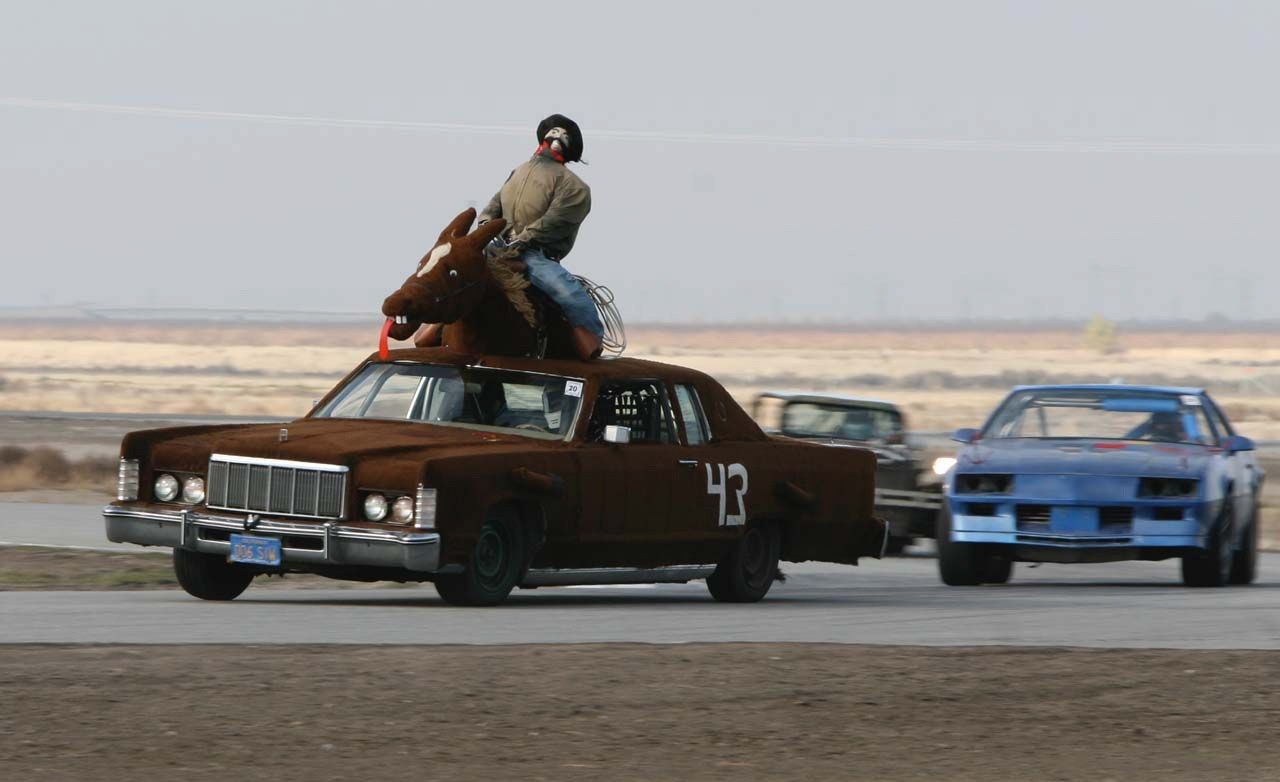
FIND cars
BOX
[740,392,952,561]
[101,346,893,607]
[934,381,1267,590]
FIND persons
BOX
[539,380,595,441]
[1143,411,1197,444]
[843,412,877,440]
[413,114,606,358]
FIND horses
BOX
[379,206,627,359]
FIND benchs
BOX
[480,412,548,431]
